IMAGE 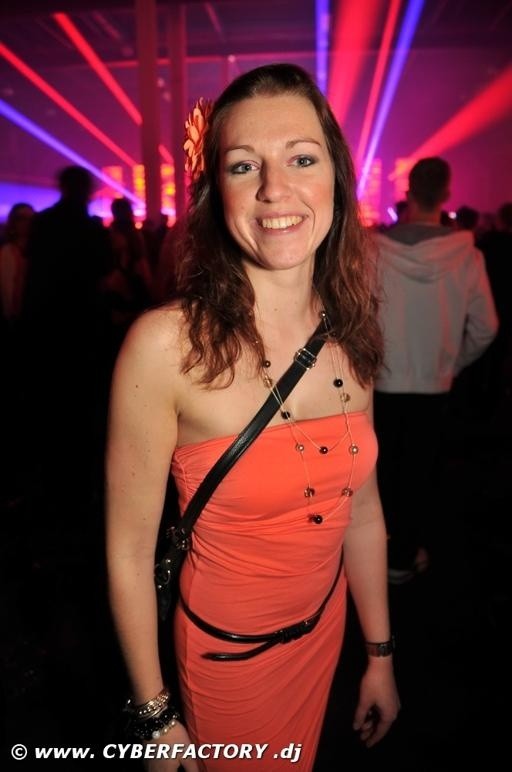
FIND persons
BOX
[105,60,403,771]
[0,153,511,586]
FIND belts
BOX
[179,547,347,667]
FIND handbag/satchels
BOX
[152,471,192,732]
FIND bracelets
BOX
[121,687,181,742]
[365,634,395,658]
